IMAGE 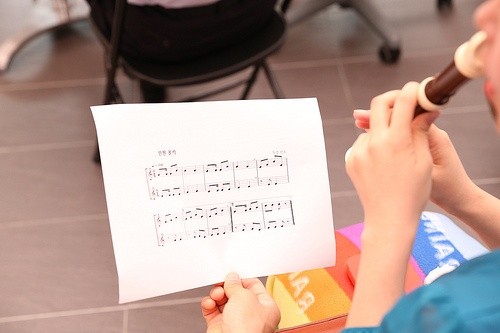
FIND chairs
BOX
[88,0,287,165]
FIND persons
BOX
[201,269,281,333]
[343,0,500,333]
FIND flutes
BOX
[388,30,490,121]
